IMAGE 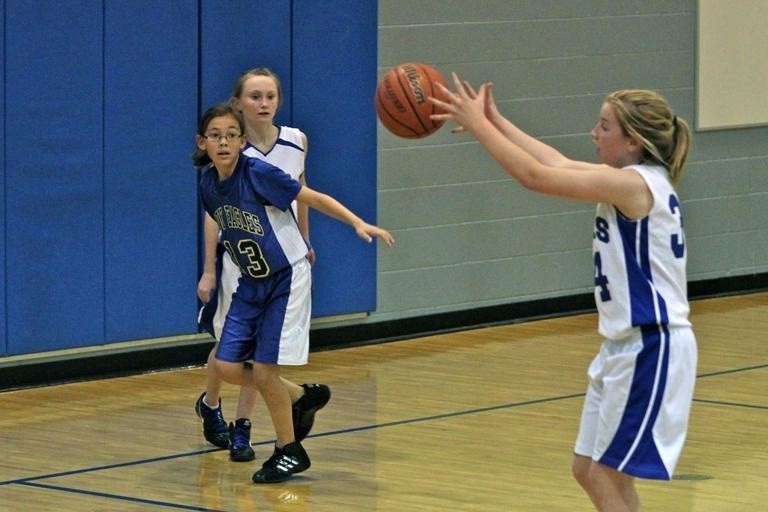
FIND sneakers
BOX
[292,384,331,441]
[252,442,311,484]
[230,418,255,462]
[195,392,229,446]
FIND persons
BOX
[195,68,315,461]
[428,73,698,512]
[191,102,396,484]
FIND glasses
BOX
[201,132,243,142]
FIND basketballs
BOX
[375,63,453,138]
